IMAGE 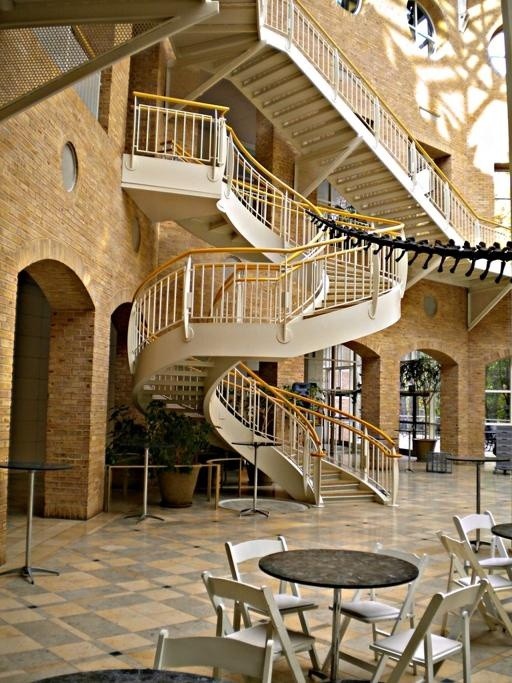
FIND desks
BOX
[121,443,175,523]
[259,549,419,683]
[30,669,237,683]
[445,456,512,551]
[231,442,283,517]
[491,523,512,547]
[0,463,74,585]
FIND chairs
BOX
[321,543,428,683]
[369,579,490,683]
[153,628,274,683]
[435,530,512,636]
[452,510,512,581]
[201,571,306,683]
[225,535,320,670]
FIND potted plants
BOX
[400,357,443,461]
[108,400,221,508]
